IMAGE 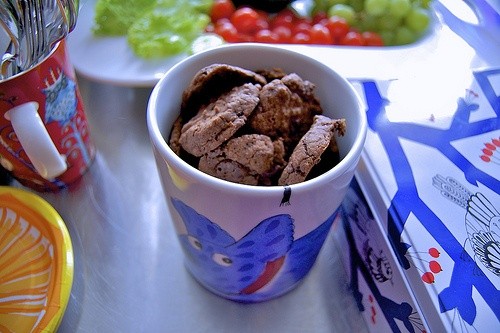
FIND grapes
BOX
[310,0,429,45]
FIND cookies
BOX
[165,62,348,187]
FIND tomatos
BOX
[206,0,384,46]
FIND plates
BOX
[64,0,444,88]
[0,185,74,332]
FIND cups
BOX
[0,28,96,194]
[147,44,367,304]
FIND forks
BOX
[0,0,79,81]
